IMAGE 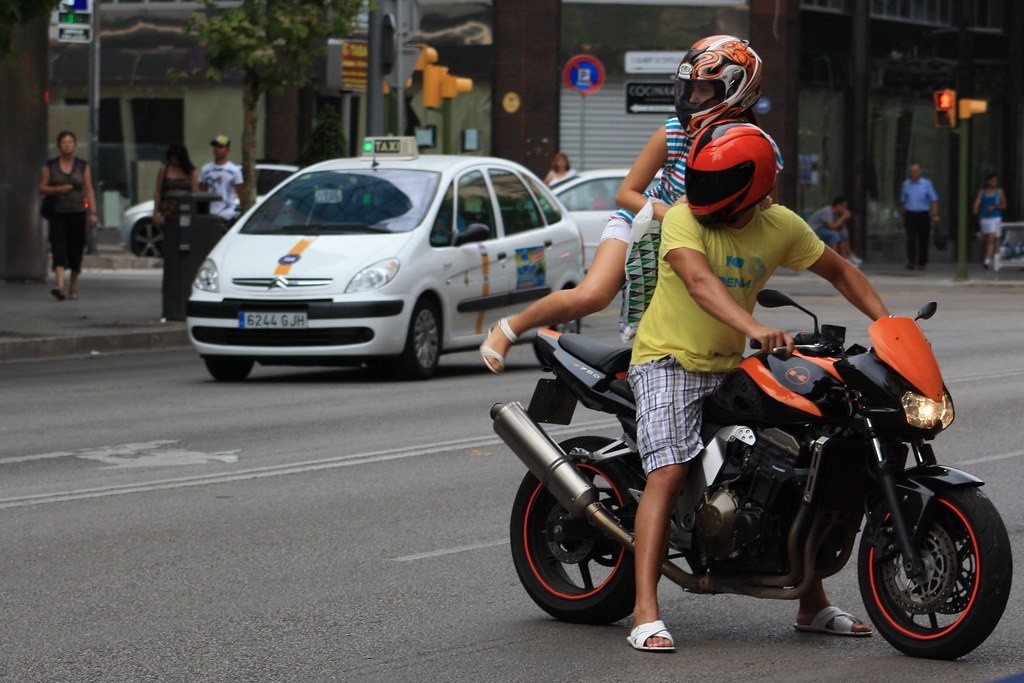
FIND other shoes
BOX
[51,288,65,300]
[69,286,78,301]
[984,258,993,270]
[905,260,915,270]
[918,261,926,270]
[845,253,864,269]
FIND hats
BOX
[210,135,231,148]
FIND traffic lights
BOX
[957,98,989,121]
[932,88,957,129]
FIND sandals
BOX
[480,317,519,375]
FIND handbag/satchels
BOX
[620,196,669,343]
[933,222,948,251]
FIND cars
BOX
[520,166,662,288]
[118,162,359,261]
[182,151,588,386]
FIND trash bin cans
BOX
[161,191,228,322]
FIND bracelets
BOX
[90,211,97,215]
[932,214,937,217]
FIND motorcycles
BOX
[488,288,1015,662]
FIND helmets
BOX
[685,117,778,225]
[673,35,763,139]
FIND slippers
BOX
[626,617,676,650]
[792,604,874,635]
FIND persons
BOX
[628,118,897,650]
[899,162,938,270]
[39,130,99,301]
[200,134,247,230]
[479,34,762,374]
[973,173,1006,270]
[153,141,200,229]
[544,153,579,185]
[807,196,863,268]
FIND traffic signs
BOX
[624,79,677,116]
